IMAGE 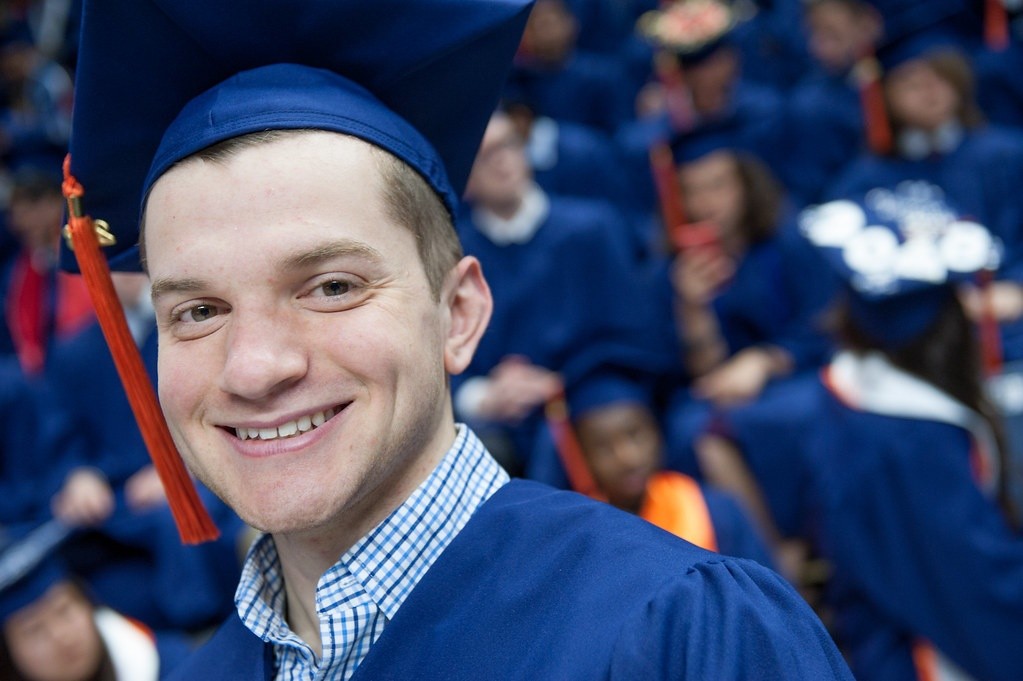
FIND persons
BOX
[0,0,1023,681]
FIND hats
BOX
[0,0,1023,619]
[62,0,534,547]
